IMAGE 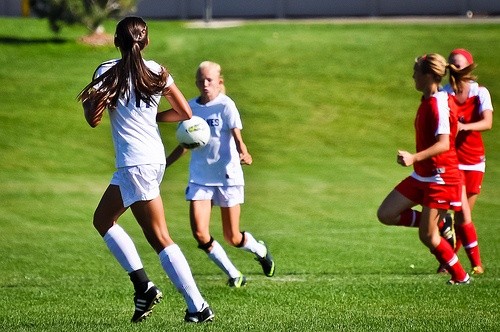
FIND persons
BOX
[166,60,276,287]
[377,53,478,286]
[76,17,215,324]
[438,49,493,274]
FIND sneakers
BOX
[255,239,275,277]
[226,271,247,287]
[471,266,484,274]
[131,281,163,323]
[447,272,470,285]
[436,264,448,274]
[184,301,215,323]
[440,214,456,249]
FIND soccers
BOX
[176,115,210,150]
